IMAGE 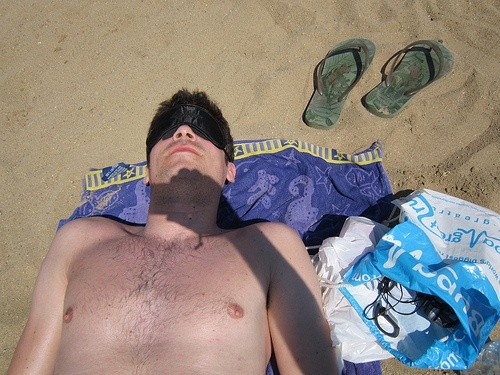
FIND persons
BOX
[6,87,341,375]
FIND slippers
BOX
[302,38,376,130]
[365,39,454,119]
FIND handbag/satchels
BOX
[338,187,500,371]
[312,216,395,363]
